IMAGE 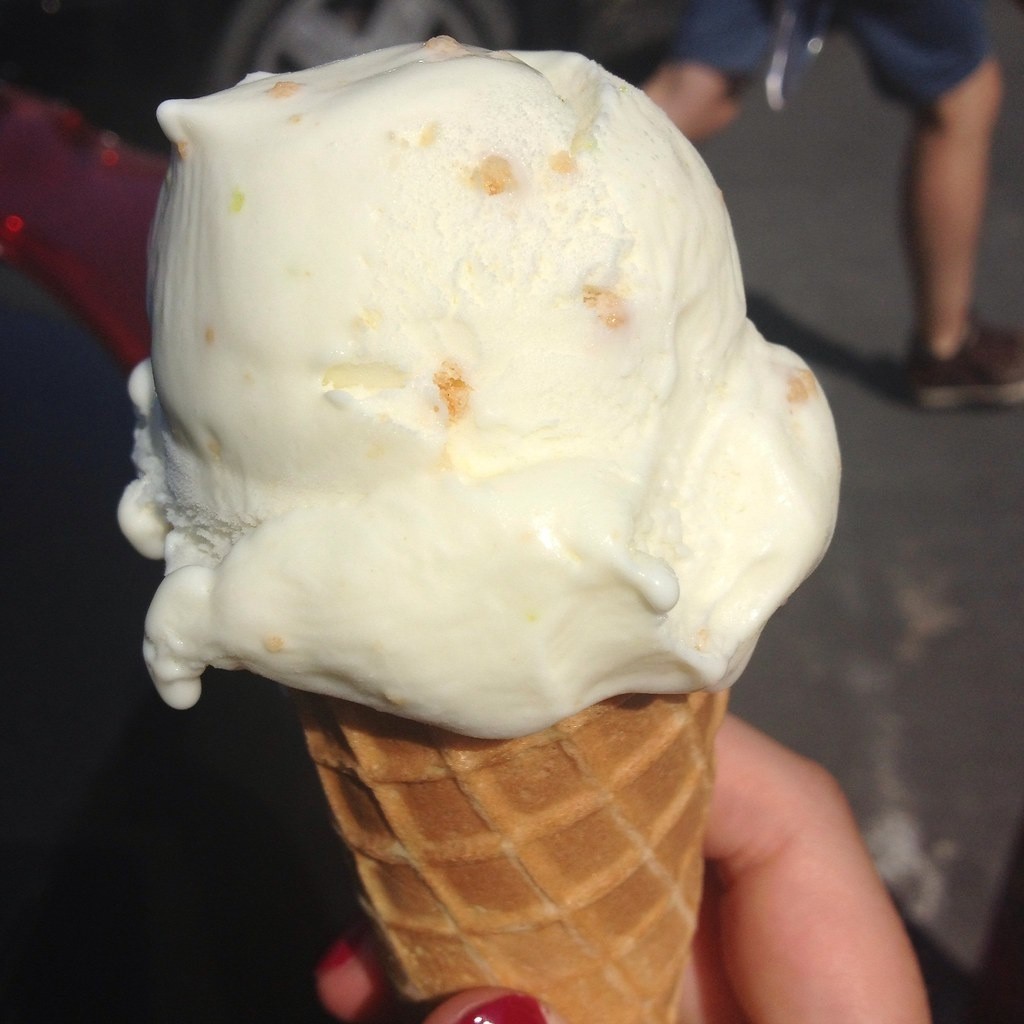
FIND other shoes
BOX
[907,315,1024,410]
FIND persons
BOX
[641,0,1024,407]
[316,711,931,1024]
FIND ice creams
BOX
[119,36,842,1023]
